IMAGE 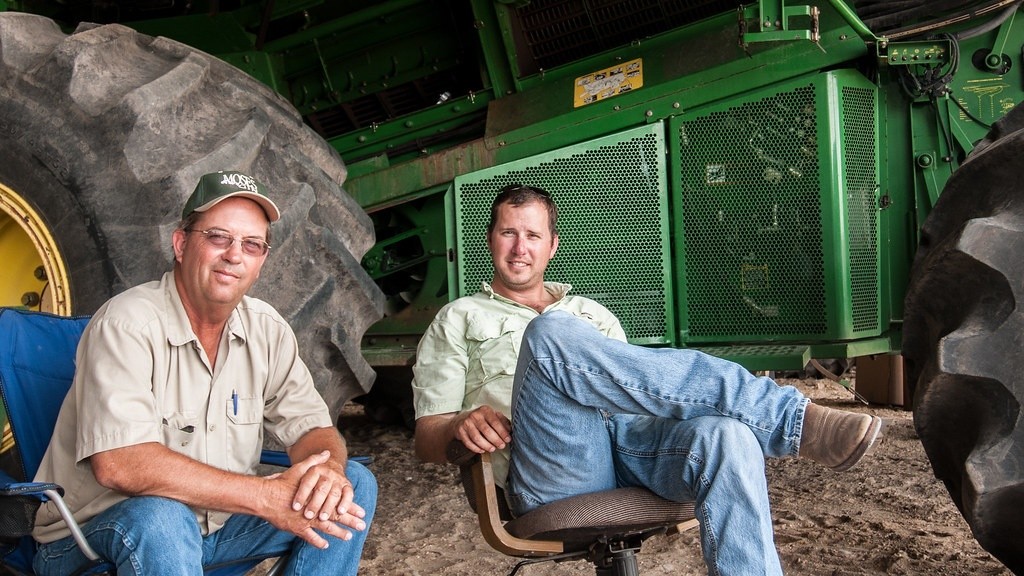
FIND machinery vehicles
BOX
[0,0,1024,576]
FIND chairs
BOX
[3,304,372,572]
[445,406,699,576]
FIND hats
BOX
[182,170,280,226]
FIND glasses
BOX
[184,229,271,256]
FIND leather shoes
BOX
[798,401,880,471]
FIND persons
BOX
[410,186,883,576]
[29,171,378,576]
